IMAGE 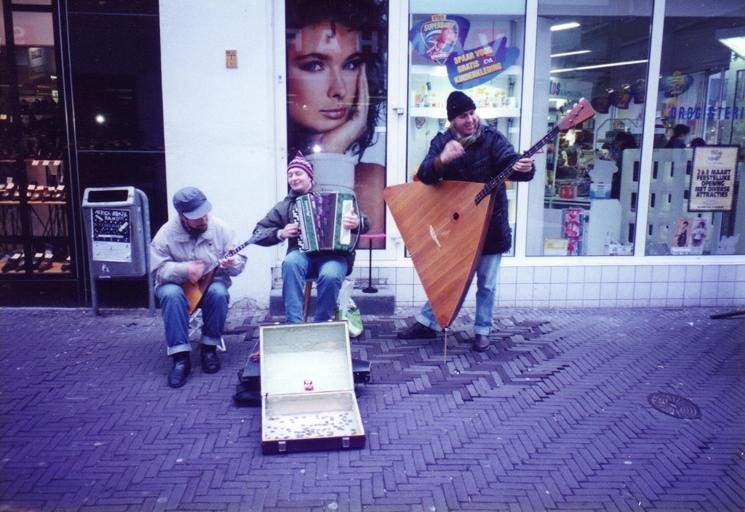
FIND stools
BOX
[166,308,226,356]
[303,277,340,322]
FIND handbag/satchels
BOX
[336,280,364,338]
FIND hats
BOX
[447,92,475,121]
[287,150,313,179]
[173,187,212,220]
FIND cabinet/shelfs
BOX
[0,157,70,277]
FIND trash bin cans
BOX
[82,186,146,280]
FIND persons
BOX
[396,91,536,353]
[252,155,372,325]
[150,186,247,387]
[556,124,706,195]
[285,0,386,249]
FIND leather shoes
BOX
[168,353,190,388]
[397,323,436,338]
[201,348,220,373]
[475,335,490,352]
